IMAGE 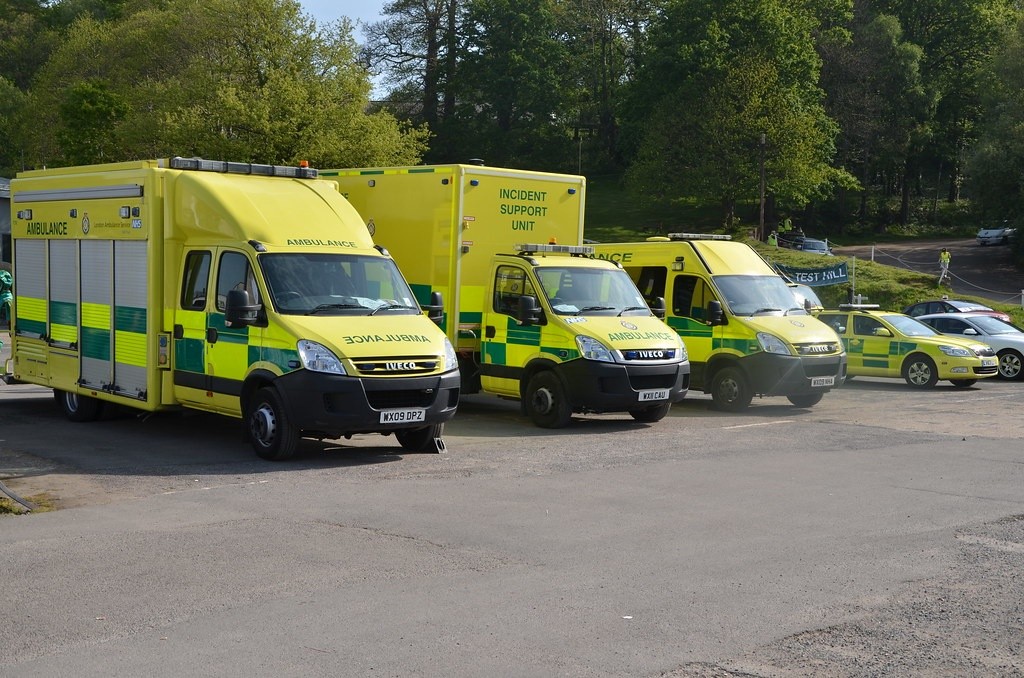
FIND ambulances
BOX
[314,164,689,429]
[811,303,999,389]
[0,155,461,461]
[584,233,848,413]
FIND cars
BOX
[893,313,1024,381]
[792,238,835,257]
[777,230,804,247]
[976,220,1018,246]
[901,300,1011,323]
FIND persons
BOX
[767,230,779,250]
[778,215,793,239]
[937,248,952,285]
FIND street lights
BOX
[757,128,768,242]
